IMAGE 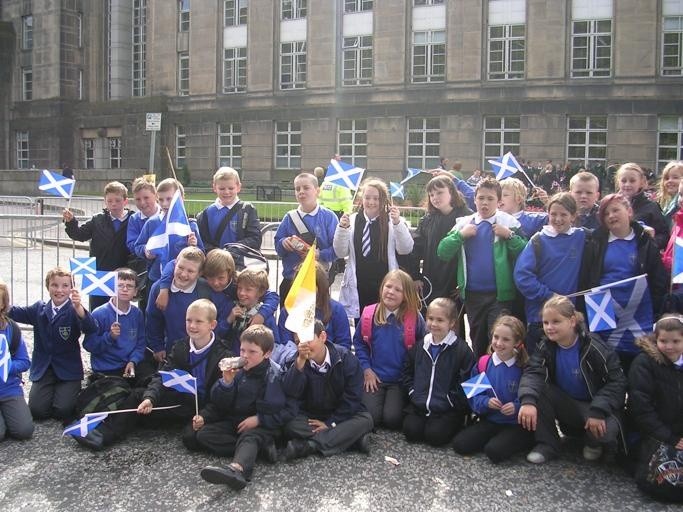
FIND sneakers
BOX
[582,446,600,460]
[527,444,548,464]
[261,440,310,464]
[356,434,371,452]
[71,428,104,450]
[201,465,246,489]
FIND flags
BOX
[669,233,683,285]
[156,367,196,398]
[324,159,365,193]
[60,412,111,440]
[145,193,192,257]
[283,239,316,344]
[67,256,96,276]
[388,180,406,200]
[579,290,618,334]
[82,269,118,299]
[406,167,421,178]
[458,371,492,401]
[589,270,657,358]
[36,169,76,199]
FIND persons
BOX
[435,176,529,360]
[331,178,415,330]
[80,265,151,385]
[143,244,232,362]
[213,266,279,358]
[313,166,324,188]
[437,151,658,209]
[153,247,280,348]
[566,170,602,231]
[613,161,669,253]
[627,312,682,453]
[577,192,665,314]
[132,177,204,311]
[68,297,235,451]
[350,269,429,428]
[659,178,681,290]
[3,267,100,421]
[194,165,262,269]
[655,162,681,222]
[401,297,475,447]
[60,161,77,202]
[61,180,140,311]
[275,257,353,352]
[124,176,164,262]
[406,176,475,343]
[194,323,289,488]
[0,283,34,442]
[516,294,627,466]
[272,171,341,309]
[495,176,550,241]
[452,314,533,463]
[277,317,375,460]
[512,191,656,354]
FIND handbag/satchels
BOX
[635,435,683,504]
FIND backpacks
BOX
[76,372,131,421]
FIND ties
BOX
[362,222,371,257]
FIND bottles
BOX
[219,355,249,373]
